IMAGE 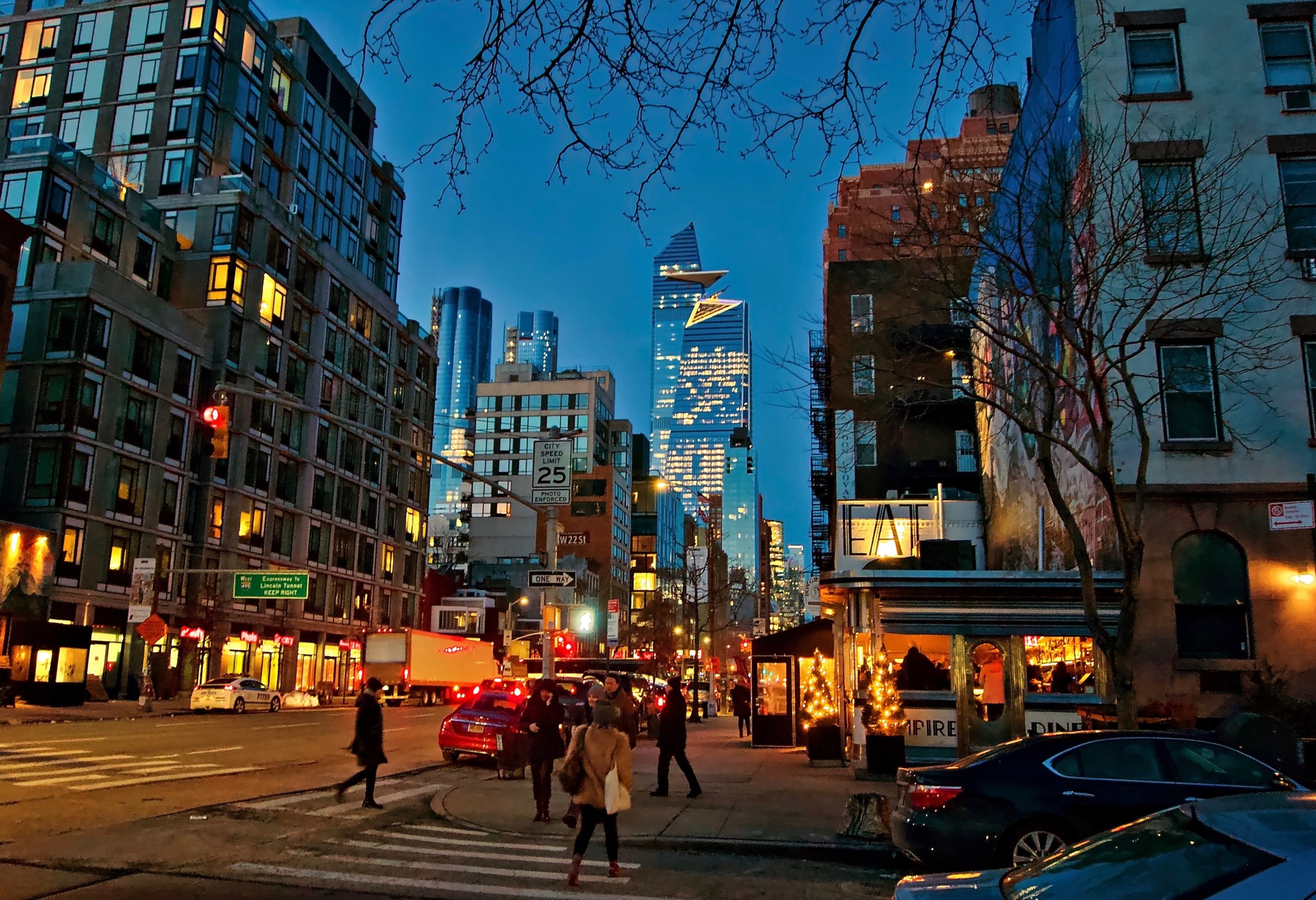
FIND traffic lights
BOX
[567,606,595,635]
[203,405,228,458]
[741,640,751,650]
[541,606,560,633]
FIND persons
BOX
[730,678,752,737]
[334,677,388,810]
[979,654,1005,721]
[520,678,565,822]
[898,646,951,691]
[1026,661,1095,693]
[650,675,702,799]
[566,700,632,890]
[562,673,636,829]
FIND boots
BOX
[567,853,582,886]
[608,861,624,877]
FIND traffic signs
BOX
[527,570,576,588]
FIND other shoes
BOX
[332,783,342,802]
[362,798,383,810]
[686,788,703,799]
[534,812,542,821]
[543,812,551,821]
[562,814,577,829]
[649,790,668,796]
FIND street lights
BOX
[652,479,715,715]
[505,597,528,667]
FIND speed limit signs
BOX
[532,440,572,488]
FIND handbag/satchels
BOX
[604,760,631,815]
[557,727,588,796]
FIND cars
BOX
[686,680,723,712]
[891,793,1316,900]
[189,673,283,714]
[889,729,1316,874]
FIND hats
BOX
[587,684,605,701]
[591,700,620,724]
[667,675,681,690]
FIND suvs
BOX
[439,669,687,771]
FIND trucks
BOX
[361,626,503,707]
